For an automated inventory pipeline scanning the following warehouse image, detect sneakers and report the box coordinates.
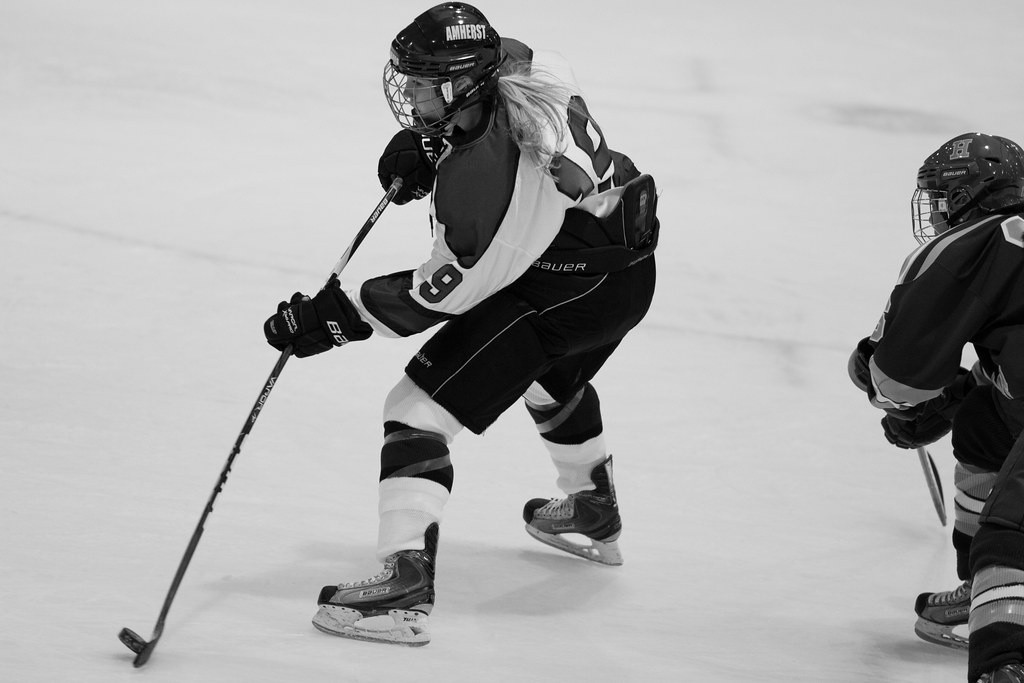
[912,576,973,652]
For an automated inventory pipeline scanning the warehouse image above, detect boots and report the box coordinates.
[520,454,625,569]
[312,521,440,644]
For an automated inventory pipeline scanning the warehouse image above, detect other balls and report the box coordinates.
[118,628,145,653]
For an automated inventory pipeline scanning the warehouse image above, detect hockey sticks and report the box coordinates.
[917,445,947,526]
[134,174,403,668]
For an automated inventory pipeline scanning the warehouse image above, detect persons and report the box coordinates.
[267,0,660,615]
[848,130,1024,683]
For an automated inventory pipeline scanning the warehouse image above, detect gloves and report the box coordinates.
[878,373,981,449]
[376,129,435,204]
[263,288,371,358]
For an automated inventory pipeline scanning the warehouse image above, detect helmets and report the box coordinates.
[380,2,502,134]
[910,131,1024,244]
